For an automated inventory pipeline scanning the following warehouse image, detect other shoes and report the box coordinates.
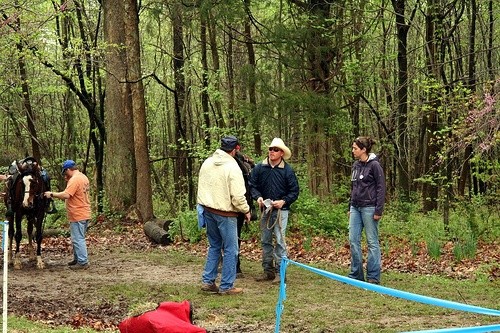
[68,260,77,265]
[218,286,243,296]
[70,263,90,271]
[200,282,218,293]
[272,273,280,283]
[256,272,276,282]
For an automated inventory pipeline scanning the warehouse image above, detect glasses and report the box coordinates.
[269,147,283,153]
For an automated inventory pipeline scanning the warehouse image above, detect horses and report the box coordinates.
[4,159,47,270]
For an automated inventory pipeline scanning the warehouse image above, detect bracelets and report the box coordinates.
[51,192,54,197]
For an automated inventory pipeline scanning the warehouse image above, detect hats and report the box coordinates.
[220,136,240,151]
[61,160,75,175]
[264,138,291,159]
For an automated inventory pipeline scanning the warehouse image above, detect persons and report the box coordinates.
[347,136,386,285]
[248,138,300,285]
[197,136,251,295]
[44,160,92,270]
